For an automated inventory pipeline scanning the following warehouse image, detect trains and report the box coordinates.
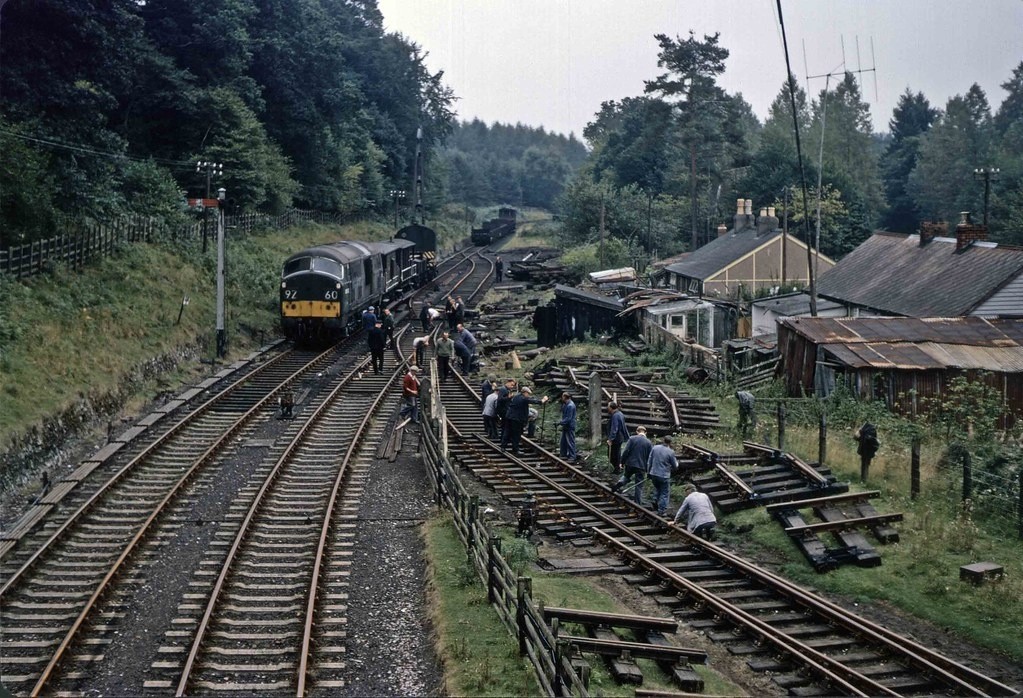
[469,206,518,246]
[277,219,439,353]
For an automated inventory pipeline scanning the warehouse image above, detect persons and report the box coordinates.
[419,306,444,333]
[647,435,679,515]
[495,257,503,283]
[612,425,652,505]
[412,335,429,367]
[607,401,630,474]
[435,331,455,382]
[454,340,471,376]
[400,365,421,424]
[667,483,716,539]
[362,305,395,375]
[446,295,465,333]
[556,391,576,461]
[457,324,477,354]
[481,373,548,455]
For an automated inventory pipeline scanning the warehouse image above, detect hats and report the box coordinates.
[411,366,419,373]
[522,387,532,394]
[369,306,375,310]
[685,484,695,491]
[376,320,383,323]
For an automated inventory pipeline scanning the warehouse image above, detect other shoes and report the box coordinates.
[702,528,708,541]
[710,529,717,541]
[400,413,404,420]
[612,482,623,492]
[412,421,421,424]
